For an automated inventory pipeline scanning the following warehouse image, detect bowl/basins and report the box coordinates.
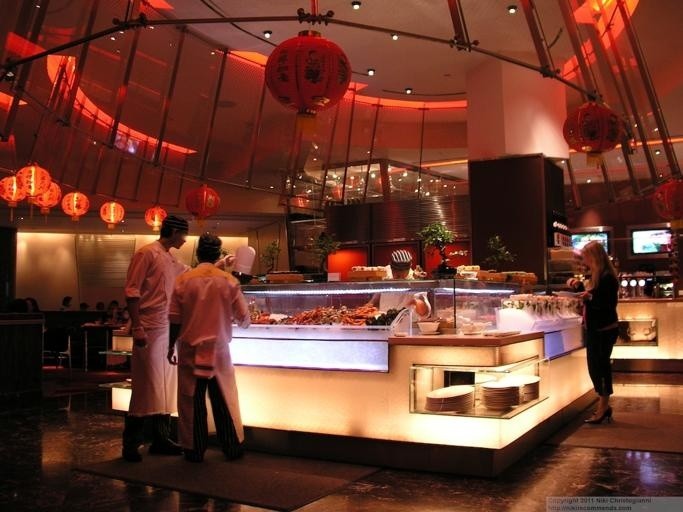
[461,271,478,277]
[418,322,440,332]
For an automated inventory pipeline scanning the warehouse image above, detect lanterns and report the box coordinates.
[61,190,90,222]
[100,201,125,229]
[145,207,167,231]
[650,179,682,221]
[562,98,623,172]
[264,31,351,118]
[15,163,51,201]
[0,175,25,208]
[33,181,61,215]
[184,184,221,227]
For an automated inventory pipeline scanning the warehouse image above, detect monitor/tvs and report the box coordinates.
[571,231,608,258]
[629,227,672,256]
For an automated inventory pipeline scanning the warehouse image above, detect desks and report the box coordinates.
[81,322,155,374]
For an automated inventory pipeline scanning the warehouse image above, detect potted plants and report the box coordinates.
[415,222,458,279]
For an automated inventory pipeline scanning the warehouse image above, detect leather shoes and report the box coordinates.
[122,447,142,461]
[150,440,183,454]
[183,451,202,461]
[227,449,245,462]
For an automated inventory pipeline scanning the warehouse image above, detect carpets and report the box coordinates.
[73,444,382,512]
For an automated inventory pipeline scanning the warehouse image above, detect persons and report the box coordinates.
[363,250,431,323]
[566,240,620,425]
[122,214,236,463]
[168,232,250,461]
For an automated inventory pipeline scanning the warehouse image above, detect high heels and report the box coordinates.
[585,405,613,424]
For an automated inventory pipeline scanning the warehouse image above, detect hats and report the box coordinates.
[391,250,413,271]
[234,246,255,275]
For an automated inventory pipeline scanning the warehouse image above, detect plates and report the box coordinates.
[418,332,440,335]
[482,383,519,409]
[552,291,582,297]
[463,277,478,282]
[519,375,540,403]
[425,385,474,413]
[484,329,522,335]
